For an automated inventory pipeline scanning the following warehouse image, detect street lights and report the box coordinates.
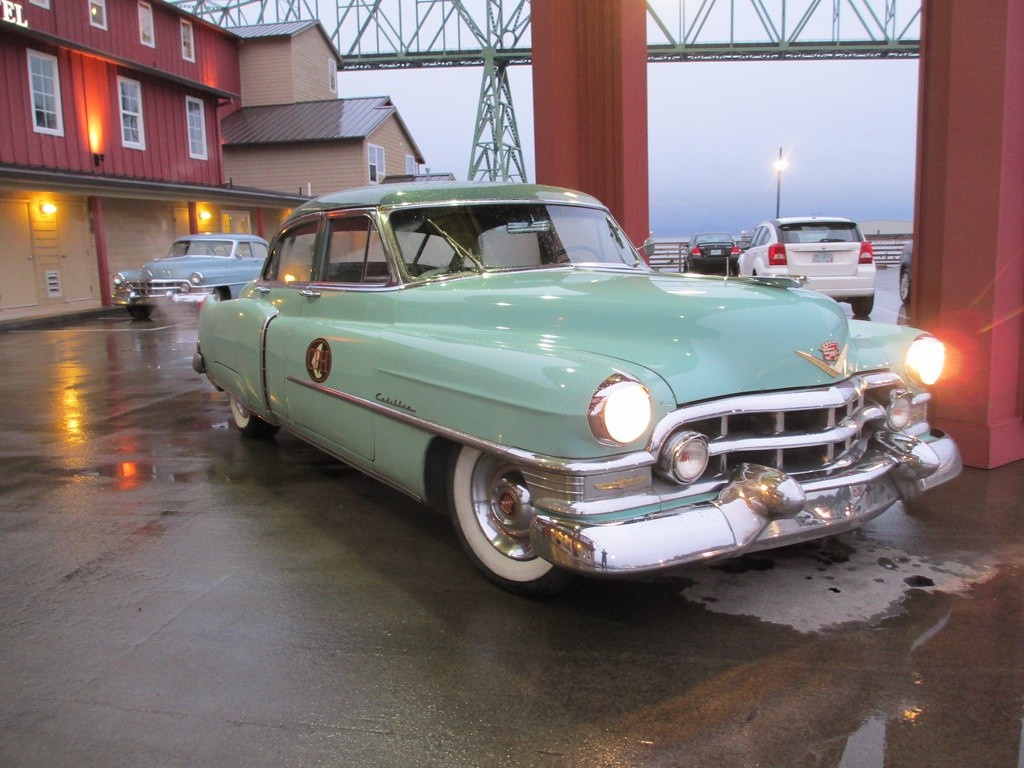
[773,147,792,218]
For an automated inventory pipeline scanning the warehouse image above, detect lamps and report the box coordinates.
[94,152,105,167]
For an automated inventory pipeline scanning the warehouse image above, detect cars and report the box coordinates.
[900,240,914,303]
[111,234,270,319]
[190,180,964,596]
[684,233,740,276]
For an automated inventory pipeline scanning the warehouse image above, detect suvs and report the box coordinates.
[738,215,877,316]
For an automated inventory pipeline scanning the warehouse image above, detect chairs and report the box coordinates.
[826,228,847,241]
[786,232,800,243]
[478,224,541,270]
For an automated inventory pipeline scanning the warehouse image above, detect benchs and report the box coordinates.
[334,261,437,283]
